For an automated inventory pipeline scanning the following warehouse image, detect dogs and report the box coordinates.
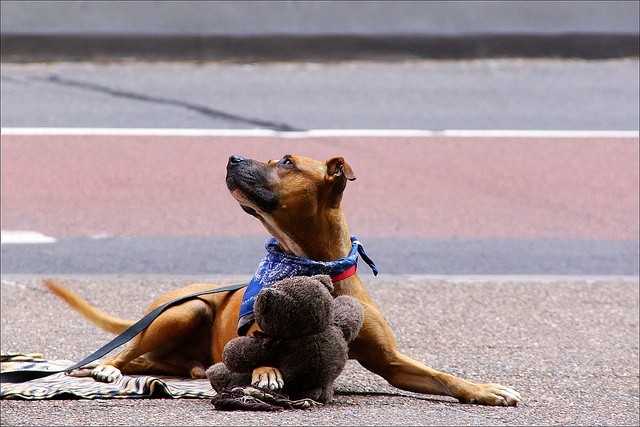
[44,154,523,407]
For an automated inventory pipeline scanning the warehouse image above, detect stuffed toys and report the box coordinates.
[205,274,364,411]
